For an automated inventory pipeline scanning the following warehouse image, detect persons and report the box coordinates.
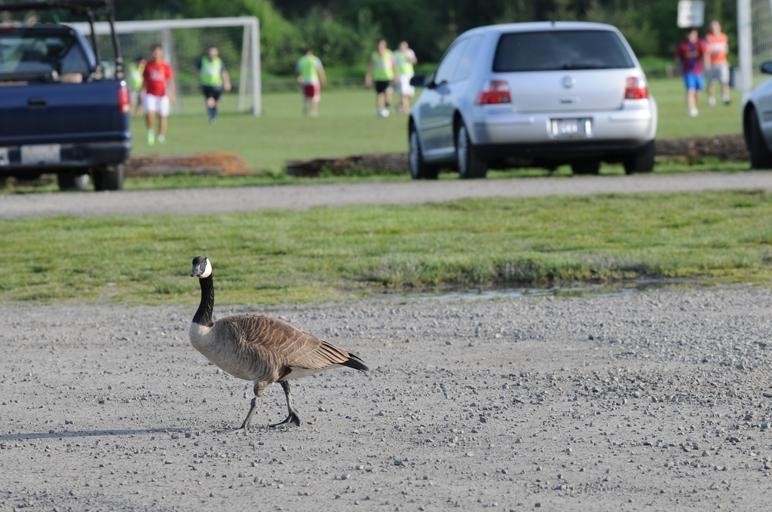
[393,40,418,113]
[126,56,146,118]
[140,44,176,146]
[196,44,232,125]
[675,25,709,116]
[296,48,327,118]
[704,18,731,106]
[365,38,399,118]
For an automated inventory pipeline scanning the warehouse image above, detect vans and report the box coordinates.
[407,21,656,179]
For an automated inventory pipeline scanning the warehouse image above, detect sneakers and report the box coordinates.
[144,128,170,148]
[685,95,738,120]
[370,101,414,118]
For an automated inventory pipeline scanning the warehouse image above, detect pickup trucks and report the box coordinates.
[1,2,129,191]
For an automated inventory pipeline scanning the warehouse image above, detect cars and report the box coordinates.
[743,58,772,170]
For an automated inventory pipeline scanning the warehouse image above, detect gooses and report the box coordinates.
[186,253,368,432]
